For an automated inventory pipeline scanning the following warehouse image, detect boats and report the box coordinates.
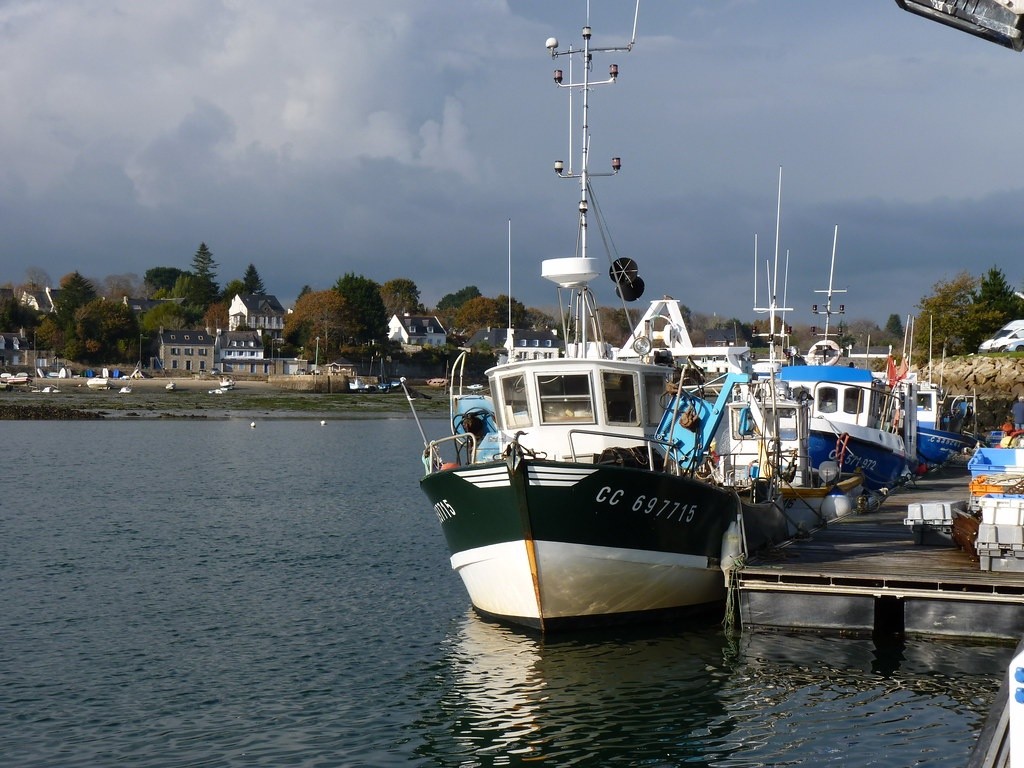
[33,365,149,394]
[426,379,450,387]
[0,377,33,392]
[418,0,977,639]
[349,377,370,393]
[466,384,484,390]
[220,377,236,390]
[210,388,228,394]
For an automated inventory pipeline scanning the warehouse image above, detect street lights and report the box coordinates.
[314,337,320,393]
[140,333,143,373]
[272,339,276,375]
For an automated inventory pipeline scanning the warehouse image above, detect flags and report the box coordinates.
[895,359,908,381]
[888,357,897,388]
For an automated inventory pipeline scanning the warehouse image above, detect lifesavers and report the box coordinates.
[807,340,841,368]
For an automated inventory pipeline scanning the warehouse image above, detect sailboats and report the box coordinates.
[155,356,176,391]
[366,356,402,392]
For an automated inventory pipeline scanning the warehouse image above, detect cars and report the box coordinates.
[296,368,308,375]
[1005,340,1024,353]
[309,369,319,375]
[210,368,222,375]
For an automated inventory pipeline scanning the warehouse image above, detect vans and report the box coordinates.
[978,319,1024,355]
[990,328,1024,353]
